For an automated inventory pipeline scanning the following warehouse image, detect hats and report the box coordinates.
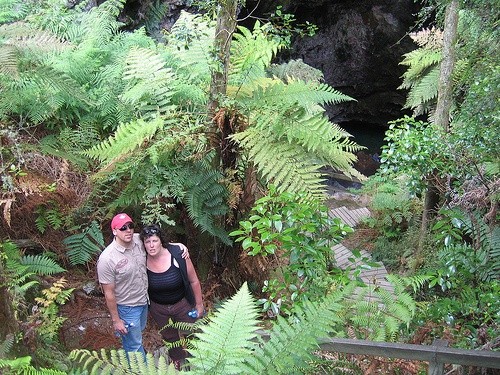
[111,213,134,231]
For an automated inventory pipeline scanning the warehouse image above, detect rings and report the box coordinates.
[186,249,188,250]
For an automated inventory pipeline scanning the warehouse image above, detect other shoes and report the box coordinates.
[174,359,182,371]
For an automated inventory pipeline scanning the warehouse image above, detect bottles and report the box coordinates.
[188,309,205,318]
[113,323,134,338]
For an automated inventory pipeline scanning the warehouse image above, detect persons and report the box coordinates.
[140,224,204,372]
[96,212,189,366]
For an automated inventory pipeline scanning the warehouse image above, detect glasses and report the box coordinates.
[142,229,159,235]
[115,223,135,231]
